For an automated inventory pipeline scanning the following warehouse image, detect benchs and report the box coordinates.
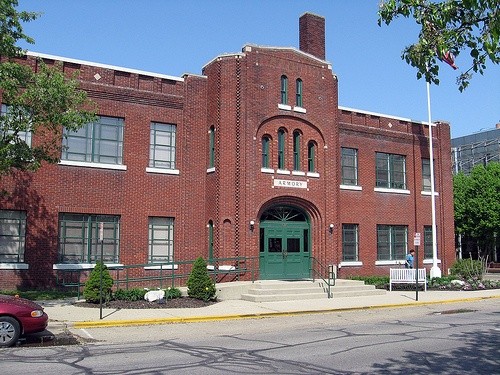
[390,268,427,292]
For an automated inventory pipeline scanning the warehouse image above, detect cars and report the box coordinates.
[0,293,48,350]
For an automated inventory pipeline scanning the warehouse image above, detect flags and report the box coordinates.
[443,51,458,71]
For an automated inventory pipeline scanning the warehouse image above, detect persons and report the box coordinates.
[405,249,415,269]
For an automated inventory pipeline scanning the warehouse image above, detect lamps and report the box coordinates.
[250,220,254,229]
[329,224,334,232]
